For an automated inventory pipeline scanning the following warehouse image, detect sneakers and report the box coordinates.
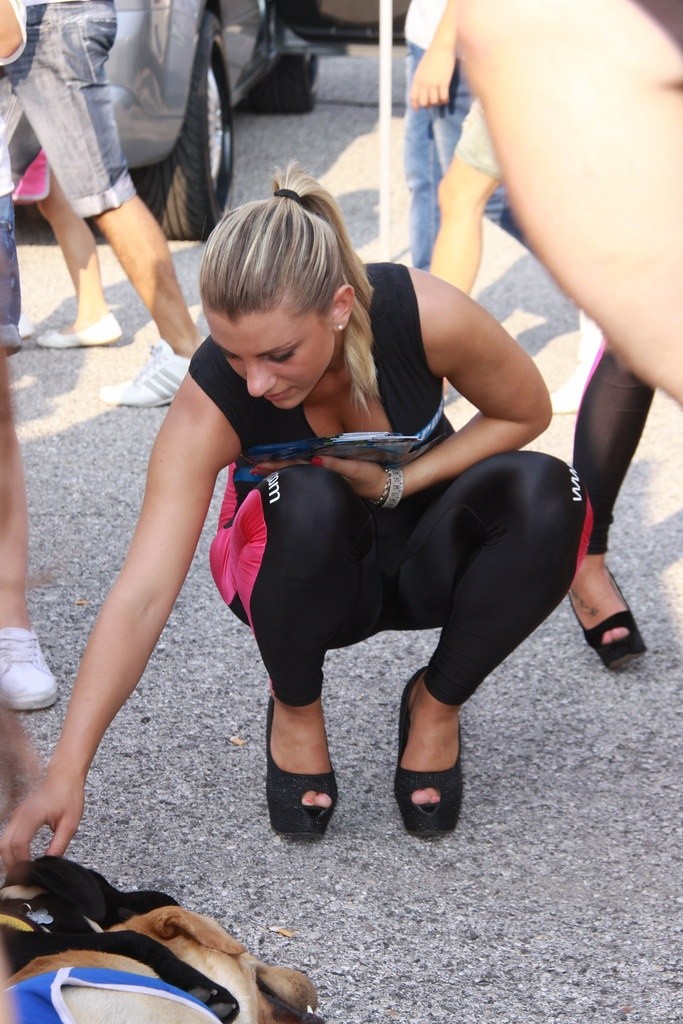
[0,626,58,710]
[99,336,191,407]
[35,312,123,348]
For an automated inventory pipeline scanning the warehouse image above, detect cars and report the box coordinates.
[0,0,322,244]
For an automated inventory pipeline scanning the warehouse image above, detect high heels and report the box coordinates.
[565,563,647,669]
[394,666,463,837]
[267,695,338,838]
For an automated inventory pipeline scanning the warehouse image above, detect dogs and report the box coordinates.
[0,906,319,1024]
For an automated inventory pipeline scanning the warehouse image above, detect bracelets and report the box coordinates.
[371,467,404,509]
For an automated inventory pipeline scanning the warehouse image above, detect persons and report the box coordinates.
[0,0,204,711]
[0,198,594,874]
[404,0,683,670]
